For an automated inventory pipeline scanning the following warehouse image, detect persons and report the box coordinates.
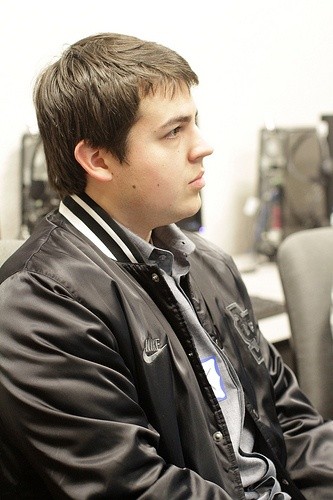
[1,33,333,500]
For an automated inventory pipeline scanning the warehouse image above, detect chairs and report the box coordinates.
[277,226,333,423]
